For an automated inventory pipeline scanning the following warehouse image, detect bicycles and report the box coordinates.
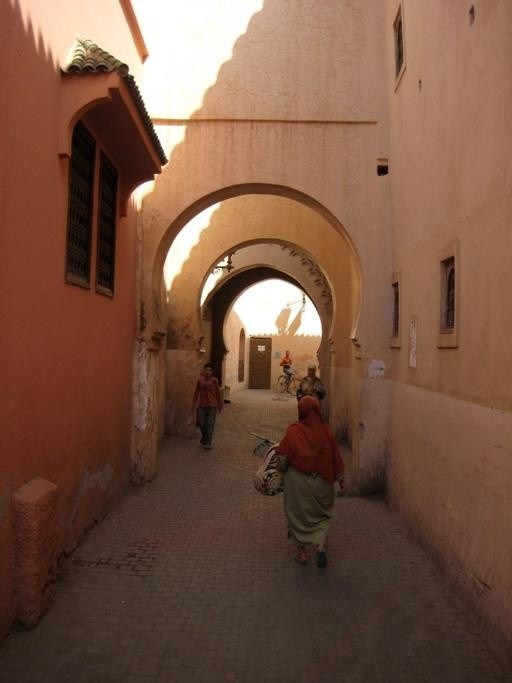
[276,368,303,396]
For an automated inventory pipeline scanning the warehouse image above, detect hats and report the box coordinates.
[308,364,316,370]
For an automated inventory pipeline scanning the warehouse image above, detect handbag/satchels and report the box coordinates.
[253,443,285,497]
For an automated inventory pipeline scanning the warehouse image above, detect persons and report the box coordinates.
[280,349,296,393]
[190,362,224,449]
[275,395,349,568]
[295,362,327,421]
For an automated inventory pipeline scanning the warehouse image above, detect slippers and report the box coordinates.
[295,553,308,564]
[317,550,328,568]
